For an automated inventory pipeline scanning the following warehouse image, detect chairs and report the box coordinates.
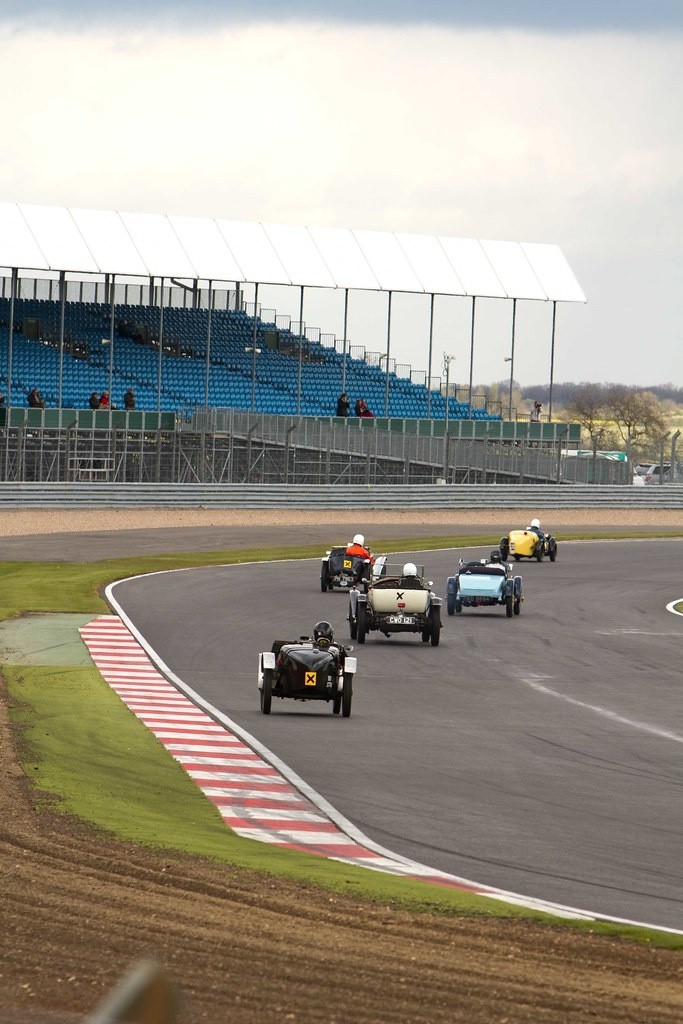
[0,298,503,420]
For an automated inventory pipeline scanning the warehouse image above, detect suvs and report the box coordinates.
[633,462,683,485]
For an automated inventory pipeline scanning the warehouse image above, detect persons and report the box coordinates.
[530,401,543,422]
[89,393,100,409]
[99,391,109,408]
[27,389,44,407]
[124,388,135,409]
[311,622,348,667]
[355,399,374,417]
[0,391,4,407]
[485,551,507,578]
[398,563,420,586]
[528,519,546,542]
[336,393,349,416]
[346,534,371,559]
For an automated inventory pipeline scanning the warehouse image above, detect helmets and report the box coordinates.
[313,621,334,644]
[403,563,417,576]
[353,533,364,547]
[531,519,541,529]
[491,551,501,560]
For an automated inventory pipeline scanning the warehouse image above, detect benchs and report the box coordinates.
[374,577,425,590]
[460,567,506,575]
